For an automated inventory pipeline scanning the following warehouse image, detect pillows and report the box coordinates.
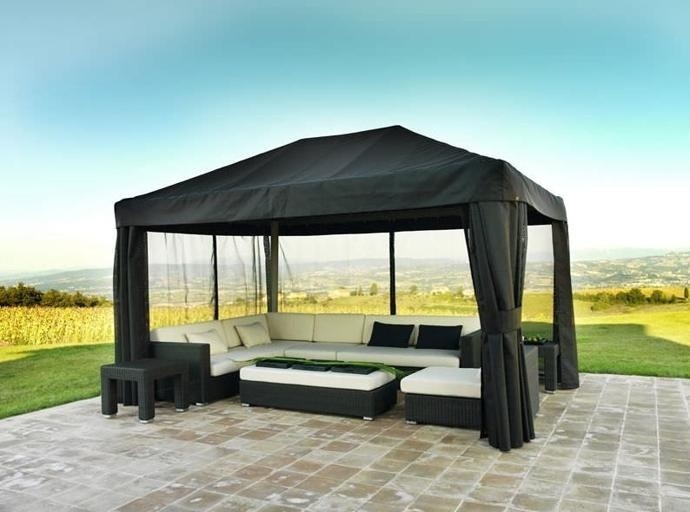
[416,322,463,350]
[234,319,272,348]
[183,328,228,356]
[366,320,416,349]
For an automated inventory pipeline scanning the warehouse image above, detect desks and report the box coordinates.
[97,357,194,424]
[525,338,560,392]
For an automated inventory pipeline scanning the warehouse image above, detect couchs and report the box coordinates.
[148,308,481,407]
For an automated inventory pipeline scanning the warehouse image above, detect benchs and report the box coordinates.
[237,359,398,423]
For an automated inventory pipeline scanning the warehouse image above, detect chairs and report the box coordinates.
[399,346,543,429]
